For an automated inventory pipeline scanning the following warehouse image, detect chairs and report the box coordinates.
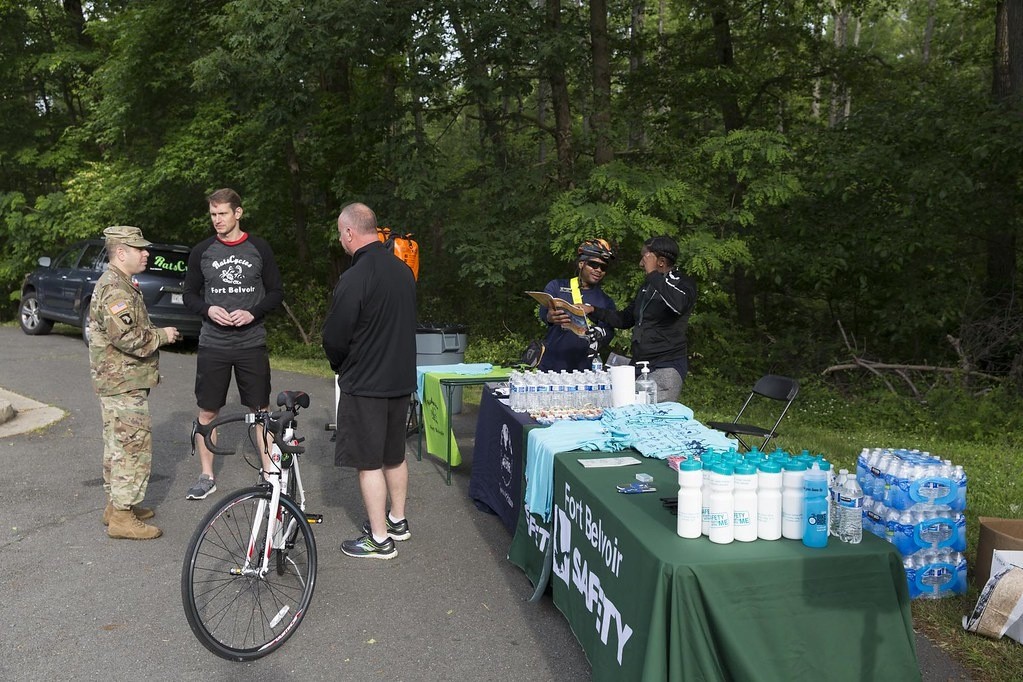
[707,373,802,450]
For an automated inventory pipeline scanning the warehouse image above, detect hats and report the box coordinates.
[103,226,153,248]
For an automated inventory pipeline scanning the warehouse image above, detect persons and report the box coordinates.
[321,202,418,561]
[571,235,698,405]
[534,236,618,376]
[182,187,285,500]
[87,226,180,540]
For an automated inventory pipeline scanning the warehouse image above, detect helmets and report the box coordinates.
[577,238,616,264]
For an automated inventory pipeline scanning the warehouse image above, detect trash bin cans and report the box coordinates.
[408,328,467,414]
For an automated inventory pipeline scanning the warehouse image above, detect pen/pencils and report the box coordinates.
[660,498,678,515]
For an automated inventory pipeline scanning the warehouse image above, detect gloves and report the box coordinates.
[585,325,606,358]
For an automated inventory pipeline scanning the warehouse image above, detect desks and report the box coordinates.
[522,423,923,682]
[472,382,601,535]
[414,363,515,485]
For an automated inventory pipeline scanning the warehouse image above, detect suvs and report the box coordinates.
[17,237,203,339]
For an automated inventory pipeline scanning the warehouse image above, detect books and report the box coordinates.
[525,290,591,338]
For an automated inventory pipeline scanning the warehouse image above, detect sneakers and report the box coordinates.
[186,473,217,500]
[363,510,411,541]
[257,466,271,486]
[340,532,398,560]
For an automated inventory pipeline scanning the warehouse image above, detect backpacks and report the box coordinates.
[376,227,419,283]
[501,340,545,372]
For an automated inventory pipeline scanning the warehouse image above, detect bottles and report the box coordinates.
[591,353,604,371]
[676,447,836,549]
[831,470,851,535]
[839,473,862,544]
[854,447,970,599]
[509,369,613,413]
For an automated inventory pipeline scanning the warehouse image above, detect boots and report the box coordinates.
[102,494,154,525]
[108,508,163,540]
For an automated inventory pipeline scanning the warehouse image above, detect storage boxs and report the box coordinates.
[977,516,1023,599]
[982,550,1023,644]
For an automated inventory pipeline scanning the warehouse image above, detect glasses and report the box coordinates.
[585,259,608,272]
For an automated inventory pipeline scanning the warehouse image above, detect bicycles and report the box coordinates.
[180,390,324,667]
